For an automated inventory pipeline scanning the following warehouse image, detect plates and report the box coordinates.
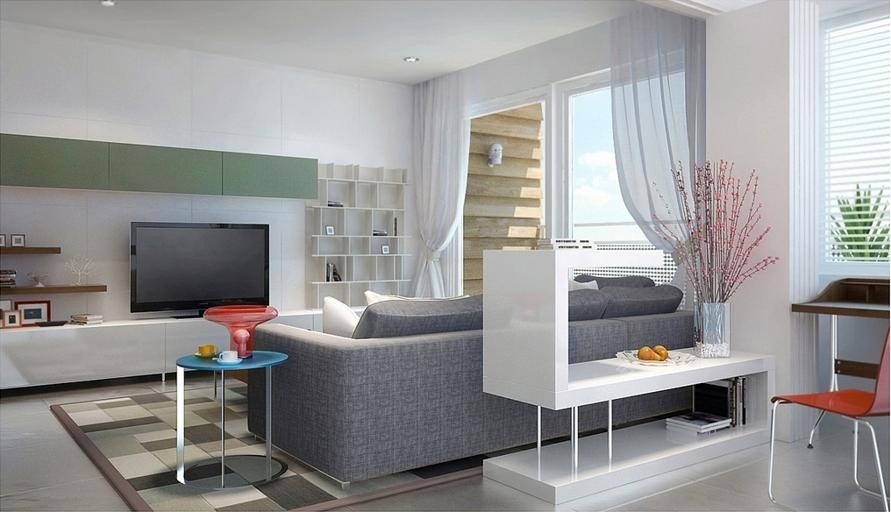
[194,351,219,357]
[217,359,242,364]
[616,351,697,371]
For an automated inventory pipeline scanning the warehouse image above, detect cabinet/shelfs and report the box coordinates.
[0,307,365,392]
[305,159,417,309]
[0,234,108,296]
[483,247,775,504]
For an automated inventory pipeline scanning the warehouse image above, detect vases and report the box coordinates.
[692,304,731,358]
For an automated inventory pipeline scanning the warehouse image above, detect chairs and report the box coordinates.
[768,324,889,512]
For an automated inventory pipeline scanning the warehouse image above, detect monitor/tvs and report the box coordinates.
[129,221,269,319]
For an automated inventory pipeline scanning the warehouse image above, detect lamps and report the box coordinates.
[488,143,504,168]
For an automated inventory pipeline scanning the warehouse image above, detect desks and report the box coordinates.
[792,278,891,450]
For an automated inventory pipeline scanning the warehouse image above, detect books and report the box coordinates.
[664,411,733,436]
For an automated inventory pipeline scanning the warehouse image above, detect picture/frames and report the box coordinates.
[381,244,391,254]
[325,225,335,235]
[1,233,7,246]
[3,312,20,328]
[15,301,51,326]
[11,234,27,247]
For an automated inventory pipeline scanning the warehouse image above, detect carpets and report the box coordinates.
[49,379,692,512]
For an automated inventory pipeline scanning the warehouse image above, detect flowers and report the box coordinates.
[647,159,781,342]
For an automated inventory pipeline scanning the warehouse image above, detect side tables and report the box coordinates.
[176,350,290,492]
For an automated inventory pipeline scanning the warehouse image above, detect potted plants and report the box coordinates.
[830,184,889,261]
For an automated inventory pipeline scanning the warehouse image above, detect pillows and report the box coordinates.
[567,277,599,291]
[364,290,471,306]
[321,296,359,337]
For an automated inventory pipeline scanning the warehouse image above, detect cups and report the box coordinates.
[219,351,238,362]
[198,345,213,354]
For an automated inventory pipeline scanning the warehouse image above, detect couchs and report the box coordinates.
[248,273,696,490]
